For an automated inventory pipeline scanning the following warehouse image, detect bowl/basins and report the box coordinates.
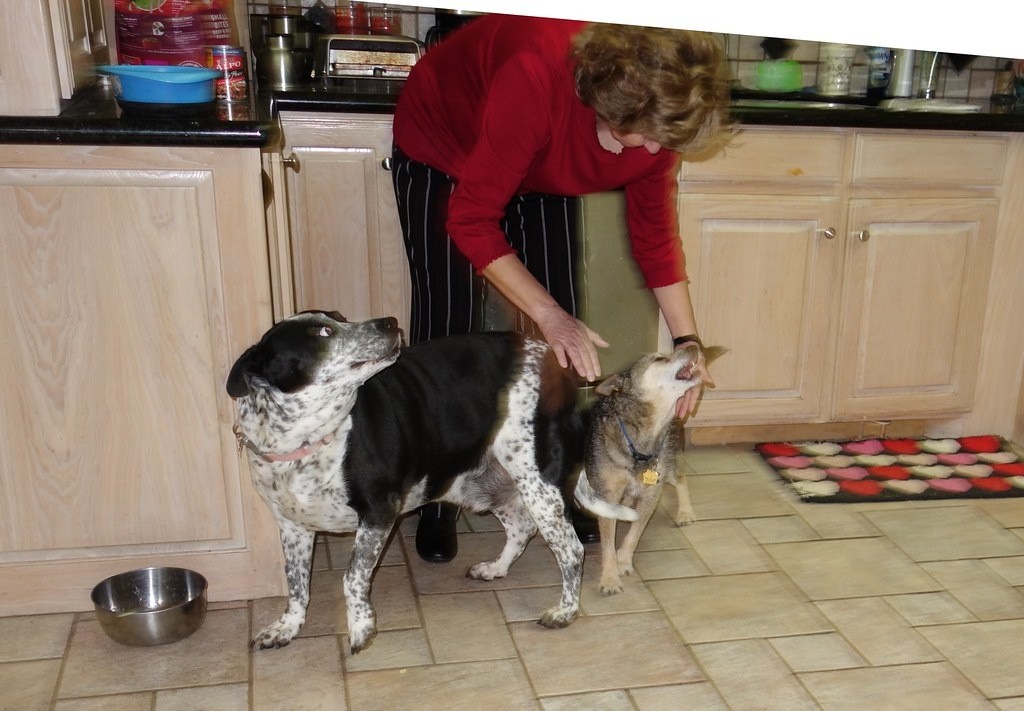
[90,566,208,651]
[94,63,224,106]
[1011,78,1023,106]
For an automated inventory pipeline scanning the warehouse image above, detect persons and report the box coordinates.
[391,13,741,563]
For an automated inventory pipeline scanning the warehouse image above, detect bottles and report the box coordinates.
[868,48,941,101]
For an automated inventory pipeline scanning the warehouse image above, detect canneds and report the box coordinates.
[211,46,249,103]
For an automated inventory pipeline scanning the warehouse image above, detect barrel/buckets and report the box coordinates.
[258,33,313,93]
[250,14,318,60]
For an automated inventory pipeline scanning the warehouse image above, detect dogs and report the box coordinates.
[225,309,641,655]
[586,344,730,596]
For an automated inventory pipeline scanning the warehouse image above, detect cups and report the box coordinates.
[267,1,299,13]
[335,1,368,33]
[820,45,856,95]
[370,4,401,34]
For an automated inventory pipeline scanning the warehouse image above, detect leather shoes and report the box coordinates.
[415,517,458,562]
[570,505,600,544]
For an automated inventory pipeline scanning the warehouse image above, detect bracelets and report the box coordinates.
[673,333,704,352]
